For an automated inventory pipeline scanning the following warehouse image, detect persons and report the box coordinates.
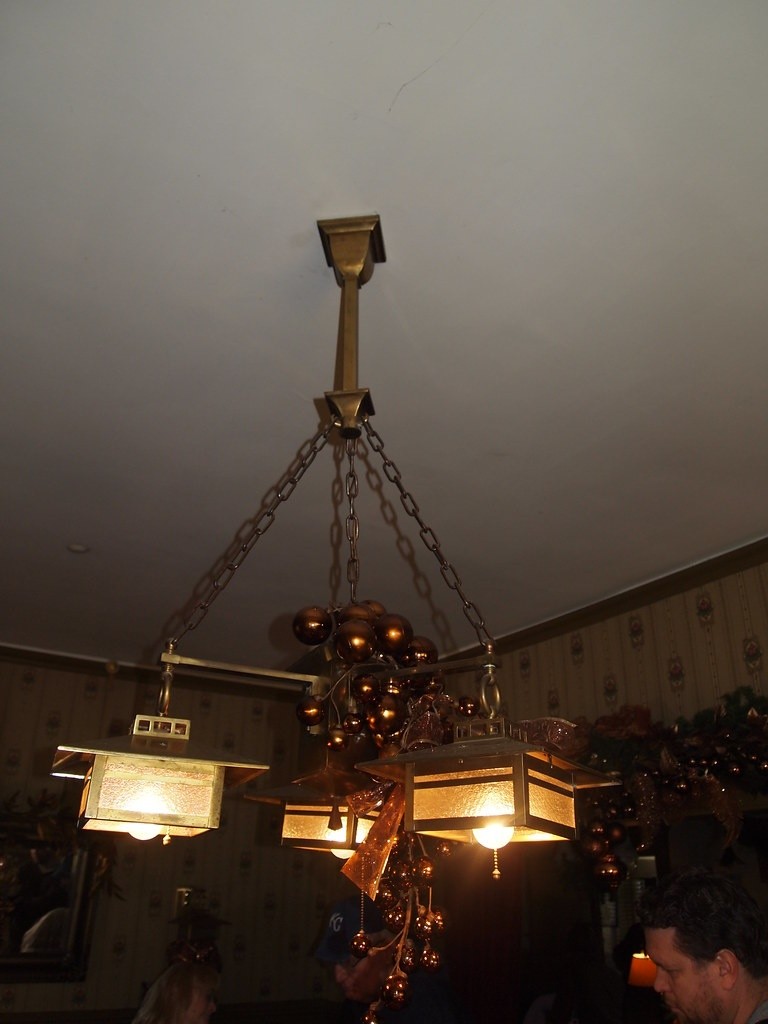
[307,895,468,1024]
[132,960,221,1024]
[635,864,768,1024]
[8,847,71,954]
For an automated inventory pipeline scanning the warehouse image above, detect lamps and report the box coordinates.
[628,952,659,988]
[48,211,623,1023]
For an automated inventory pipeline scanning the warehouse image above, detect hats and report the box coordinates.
[312,896,395,967]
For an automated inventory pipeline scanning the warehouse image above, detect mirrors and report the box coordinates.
[0,789,114,984]
